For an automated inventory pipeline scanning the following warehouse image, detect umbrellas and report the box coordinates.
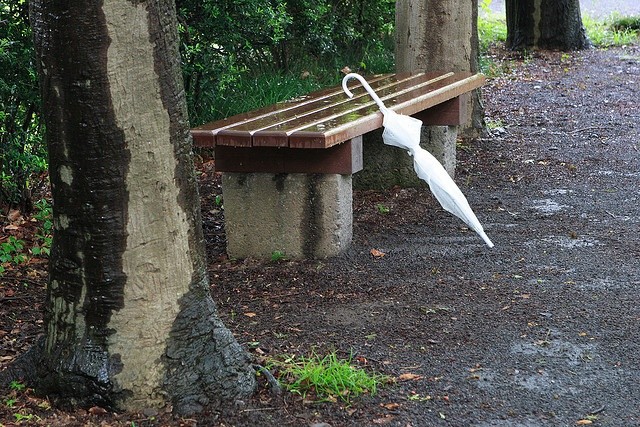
[342,72,495,248]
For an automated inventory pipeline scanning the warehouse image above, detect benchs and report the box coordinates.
[188,71,486,262]
